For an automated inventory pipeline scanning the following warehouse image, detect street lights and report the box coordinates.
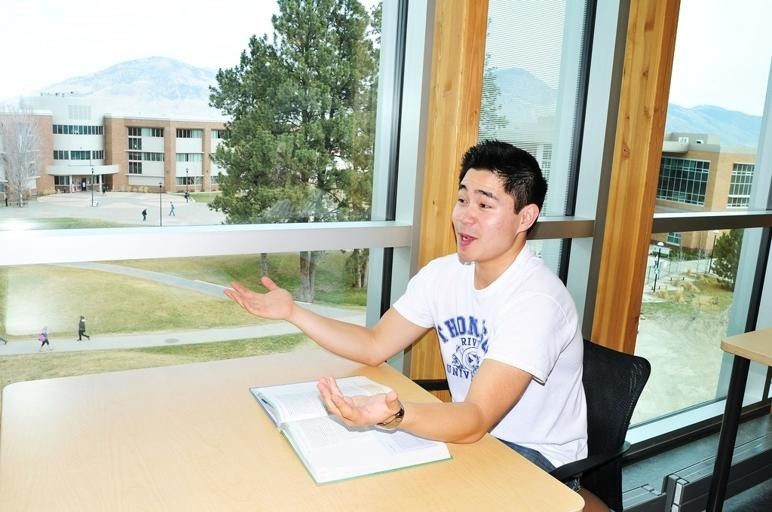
[91,169,94,206]
[652,241,664,291]
[185,168,189,202]
[708,230,719,273]
[158,182,163,227]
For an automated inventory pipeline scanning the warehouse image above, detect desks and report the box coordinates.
[706,325,772,511]
[0,347,584,511]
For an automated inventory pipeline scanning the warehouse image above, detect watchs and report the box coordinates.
[378,407,406,431]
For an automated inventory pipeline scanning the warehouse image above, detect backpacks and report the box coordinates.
[38,334,46,342]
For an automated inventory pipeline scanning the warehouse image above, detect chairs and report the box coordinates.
[411,336,651,511]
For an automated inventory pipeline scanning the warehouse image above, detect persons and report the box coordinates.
[76,315,90,342]
[0,335,8,345]
[223,138,592,497]
[141,208,147,221]
[169,201,175,216]
[184,191,190,203]
[39,324,54,353]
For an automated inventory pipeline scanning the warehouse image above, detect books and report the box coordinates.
[247,374,452,486]
